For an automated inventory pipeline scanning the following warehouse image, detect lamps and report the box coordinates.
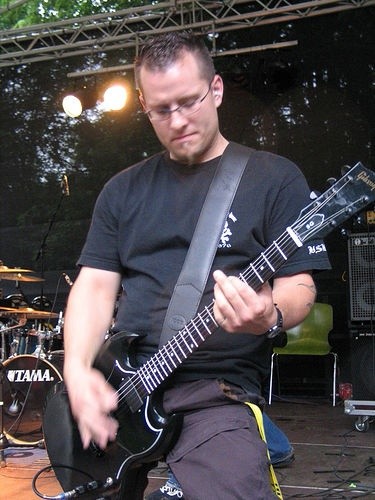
[62,73,97,119]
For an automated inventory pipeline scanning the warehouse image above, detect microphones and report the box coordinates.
[61,273,73,287]
[62,174,71,198]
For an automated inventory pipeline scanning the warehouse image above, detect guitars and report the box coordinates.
[41,161,375,500]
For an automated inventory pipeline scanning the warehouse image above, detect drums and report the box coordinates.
[9,328,64,360]
[0,354,64,446]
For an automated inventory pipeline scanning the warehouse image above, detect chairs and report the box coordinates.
[268,302,337,406]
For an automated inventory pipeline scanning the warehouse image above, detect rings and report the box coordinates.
[218,317,226,325]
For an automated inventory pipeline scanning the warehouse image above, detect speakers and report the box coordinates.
[346,231,374,322]
[350,319,375,402]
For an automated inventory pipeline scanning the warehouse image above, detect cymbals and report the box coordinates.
[0,307,58,319]
[0,265,34,273]
[0,274,47,282]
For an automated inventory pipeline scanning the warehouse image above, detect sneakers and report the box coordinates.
[148,483,183,500]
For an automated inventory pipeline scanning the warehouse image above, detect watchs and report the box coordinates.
[264,304,283,338]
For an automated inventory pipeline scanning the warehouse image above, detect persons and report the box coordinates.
[65,30,334,499]
[145,412,294,500]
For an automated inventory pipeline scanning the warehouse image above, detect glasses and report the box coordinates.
[140,80,213,121]
